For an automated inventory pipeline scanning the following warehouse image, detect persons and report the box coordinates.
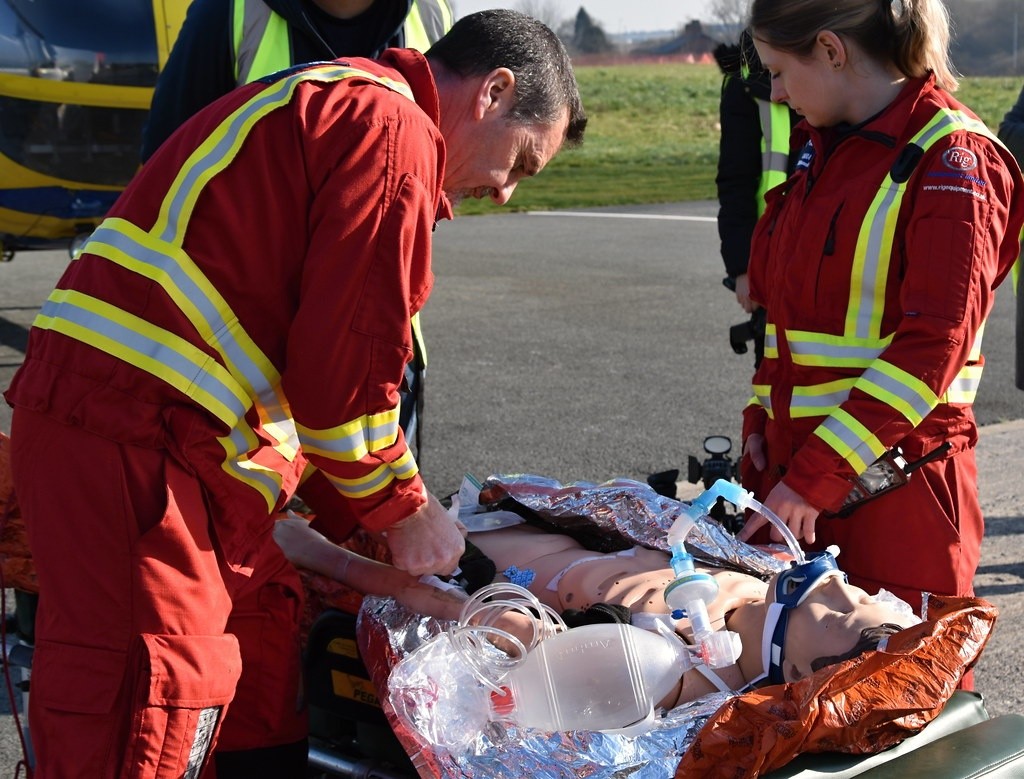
[997,87,1024,392]
[272,505,923,712]
[6,10,591,779]
[715,25,801,369]
[734,0,1024,689]
[135,1,452,473]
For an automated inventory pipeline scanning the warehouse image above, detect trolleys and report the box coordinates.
[1,590,1024,779]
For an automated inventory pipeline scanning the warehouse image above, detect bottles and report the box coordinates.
[485,623,743,731]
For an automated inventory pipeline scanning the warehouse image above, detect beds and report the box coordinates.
[0,583,1024,779]
